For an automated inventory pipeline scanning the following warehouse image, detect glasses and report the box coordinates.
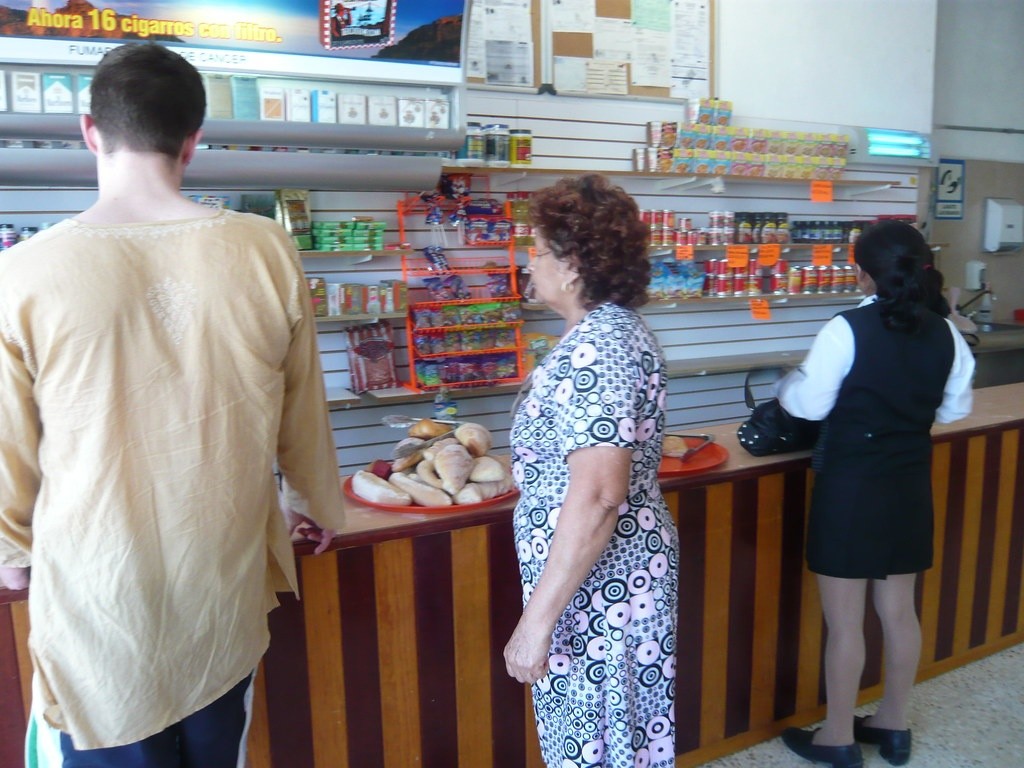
[531,249,552,257]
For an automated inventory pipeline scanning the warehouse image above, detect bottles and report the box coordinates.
[980,291,993,333]
[504,190,536,247]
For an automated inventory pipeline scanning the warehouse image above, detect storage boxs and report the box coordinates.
[633,98,851,179]
[273,188,386,251]
[307,277,408,317]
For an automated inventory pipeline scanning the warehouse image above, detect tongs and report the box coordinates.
[379,415,466,459]
[661,431,715,464]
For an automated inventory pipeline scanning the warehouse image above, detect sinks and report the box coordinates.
[974,321,1024,334]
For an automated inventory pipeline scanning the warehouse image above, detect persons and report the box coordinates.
[775,213,977,766]
[0,41,351,768]
[504,175,683,768]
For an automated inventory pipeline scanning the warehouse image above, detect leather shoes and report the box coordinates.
[853,715,911,766]
[782,727,863,768]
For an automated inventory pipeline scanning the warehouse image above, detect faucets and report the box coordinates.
[958,290,997,311]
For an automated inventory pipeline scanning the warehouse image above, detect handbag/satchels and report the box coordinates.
[737,367,822,457]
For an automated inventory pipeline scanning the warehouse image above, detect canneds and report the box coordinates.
[637,209,790,244]
[791,220,871,245]
[702,258,859,294]
[454,122,535,165]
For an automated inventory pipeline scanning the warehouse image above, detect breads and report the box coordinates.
[350,419,514,507]
[662,435,688,457]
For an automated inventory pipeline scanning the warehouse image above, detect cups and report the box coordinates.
[0,224,38,253]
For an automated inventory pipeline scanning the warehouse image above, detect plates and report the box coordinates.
[656,437,729,478]
[343,466,518,514]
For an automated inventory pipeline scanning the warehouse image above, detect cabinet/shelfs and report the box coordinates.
[395,198,525,395]
[299,248,413,409]
[366,164,901,404]
